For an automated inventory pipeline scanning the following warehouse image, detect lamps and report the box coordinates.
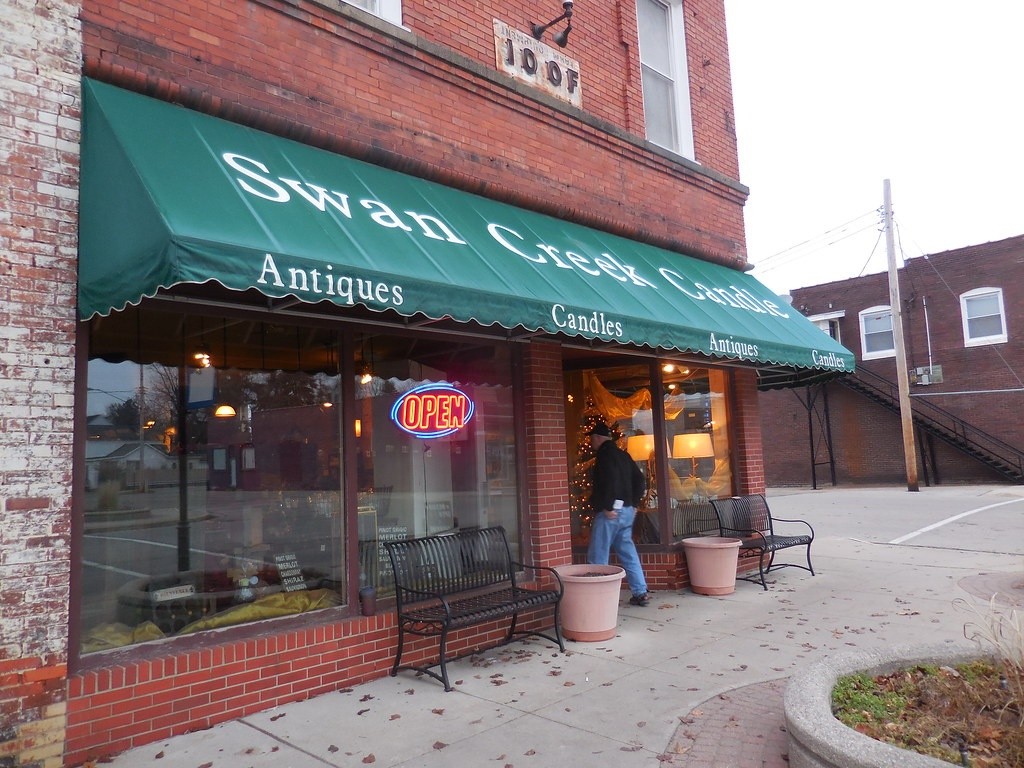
[672,433,715,480]
[532,0,573,48]
[214,308,237,417]
[628,434,673,508]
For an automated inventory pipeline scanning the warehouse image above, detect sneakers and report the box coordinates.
[630,592,649,606]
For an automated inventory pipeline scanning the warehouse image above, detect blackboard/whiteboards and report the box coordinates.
[273,552,308,592]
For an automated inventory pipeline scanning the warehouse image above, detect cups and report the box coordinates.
[361,587,377,616]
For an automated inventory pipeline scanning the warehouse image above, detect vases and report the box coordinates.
[551,564,627,642]
[681,536,743,596]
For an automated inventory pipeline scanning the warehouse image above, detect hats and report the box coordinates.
[584,423,611,437]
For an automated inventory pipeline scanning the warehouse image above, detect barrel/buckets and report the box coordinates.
[552,565,627,642]
[683,538,743,595]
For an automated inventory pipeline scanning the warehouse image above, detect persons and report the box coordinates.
[584,424,652,605]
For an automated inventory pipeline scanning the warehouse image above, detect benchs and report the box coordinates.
[383,526,566,693]
[709,494,815,591]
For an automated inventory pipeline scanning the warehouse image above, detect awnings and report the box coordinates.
[80,78,856,391]
